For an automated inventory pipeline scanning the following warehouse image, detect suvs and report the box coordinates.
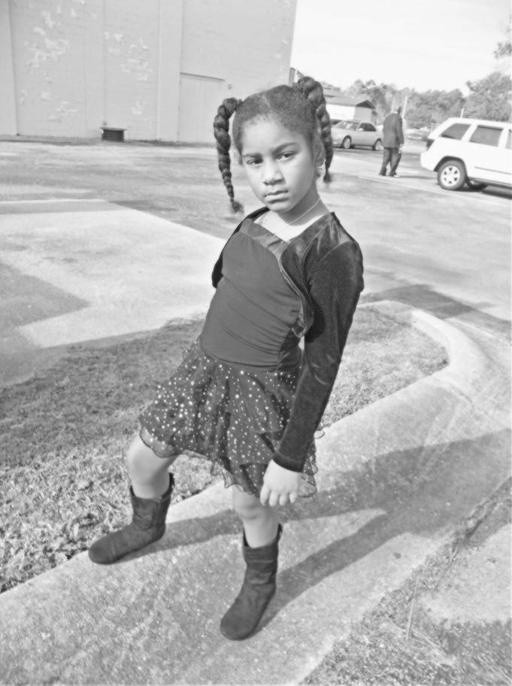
[420,115,512,193]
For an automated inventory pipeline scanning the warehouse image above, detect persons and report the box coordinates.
[378,106,404,177]
[88,77,364,641]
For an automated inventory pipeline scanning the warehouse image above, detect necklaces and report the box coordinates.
[263,197,321,233]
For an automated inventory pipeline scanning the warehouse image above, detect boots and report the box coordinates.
[220,524,282,640]
[88,474,174,563]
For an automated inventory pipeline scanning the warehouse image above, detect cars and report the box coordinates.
[331,118,384,150]
